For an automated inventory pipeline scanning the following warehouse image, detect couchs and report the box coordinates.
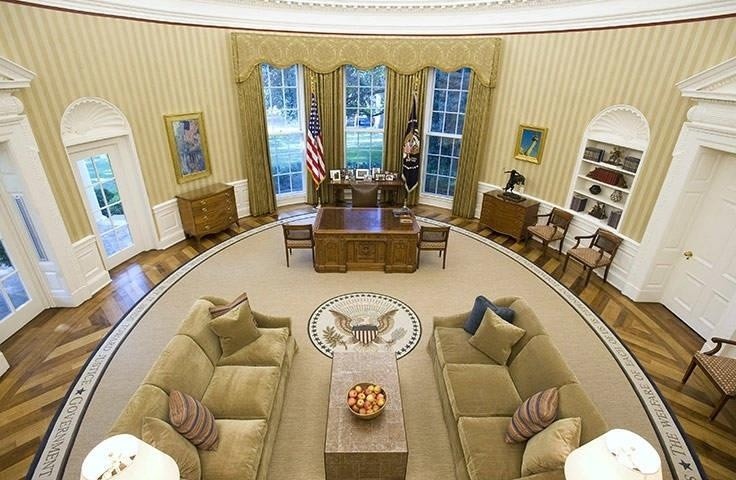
[426,295,608,480]
[103,294,299,480]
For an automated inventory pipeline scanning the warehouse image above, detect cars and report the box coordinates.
[347,116,374,127]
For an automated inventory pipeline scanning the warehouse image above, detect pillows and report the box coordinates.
[504,386,559,445]
[520,417,582,477]
[208,300,262,358]
[168,387,219,450]
[209,291,259,327]
[468,307,527,366]
[462,295,515,335]
[141,416,202,480]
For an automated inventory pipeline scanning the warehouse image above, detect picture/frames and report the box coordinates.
[329,169,397,182]
[513,124,549,165]
[163,111,212,184]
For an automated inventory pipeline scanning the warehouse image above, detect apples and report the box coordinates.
[347,383,384,414]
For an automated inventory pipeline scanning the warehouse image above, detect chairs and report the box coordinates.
[525,207,574,256]
[682,336,736,422]
[563,228,624,286]
[281,223,315,267]
[417,225,450,269]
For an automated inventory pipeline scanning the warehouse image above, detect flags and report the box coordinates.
[304,91,327,192]
[400,88,420,194]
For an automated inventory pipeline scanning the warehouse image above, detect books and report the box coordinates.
[583,146,605,162]
[607,210,622,229]
[571,195,588,212]
[623,156,640,174]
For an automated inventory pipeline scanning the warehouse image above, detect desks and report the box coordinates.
[326,178,404,207]
[312,207,420,273]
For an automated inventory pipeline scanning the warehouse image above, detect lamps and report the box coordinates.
[80,433,180,480]
[564,428,663,480]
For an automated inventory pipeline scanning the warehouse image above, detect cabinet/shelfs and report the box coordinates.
[569,139,644,230]
[478,190,541,244]
[175,182,240,245]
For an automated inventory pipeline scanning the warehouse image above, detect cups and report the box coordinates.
[345,175,349,183]
